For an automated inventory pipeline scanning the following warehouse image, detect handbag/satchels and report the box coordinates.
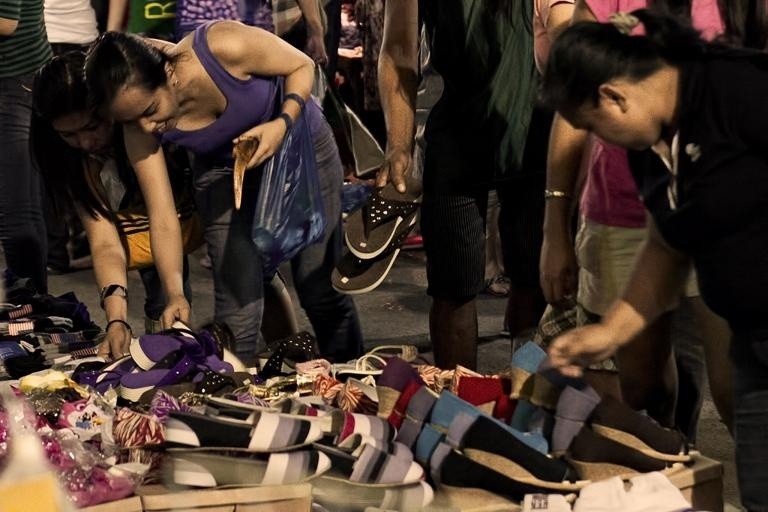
[327,84,387,179]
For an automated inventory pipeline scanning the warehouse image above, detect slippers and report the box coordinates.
[342,176,423,262]
[330,248,403,296]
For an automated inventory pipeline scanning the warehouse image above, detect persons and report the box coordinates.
[0,1,768,512]
